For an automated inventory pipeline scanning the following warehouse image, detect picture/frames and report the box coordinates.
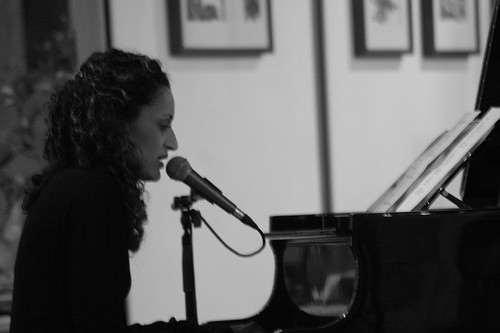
[421,0,480,56]
[167,0,274,55]
[354,0,414,54]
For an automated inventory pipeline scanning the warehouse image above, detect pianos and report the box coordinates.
[204,1,500,333]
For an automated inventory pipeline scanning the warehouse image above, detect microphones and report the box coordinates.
[166,157,258,229]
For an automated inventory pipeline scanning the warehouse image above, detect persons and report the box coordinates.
[9,49,186,333]
